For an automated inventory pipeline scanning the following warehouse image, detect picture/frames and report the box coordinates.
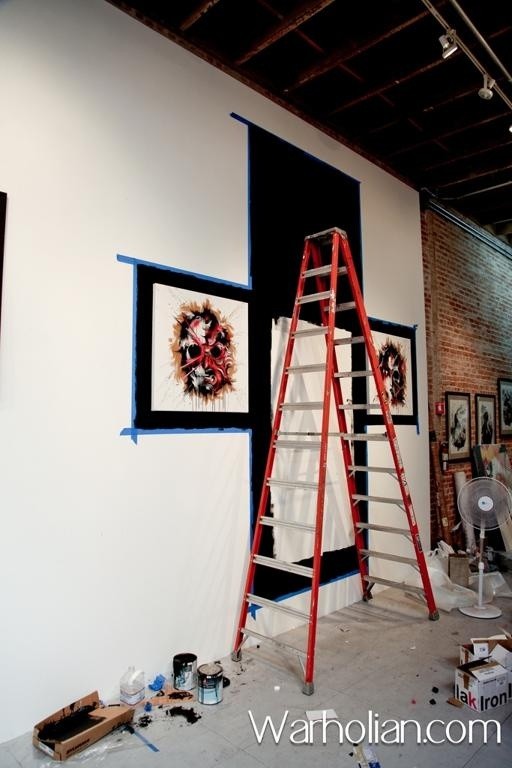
[358,315,424,428]
[133,263,261,430]
[440,375,510,467]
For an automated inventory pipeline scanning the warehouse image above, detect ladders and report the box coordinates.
[230,227,439,695]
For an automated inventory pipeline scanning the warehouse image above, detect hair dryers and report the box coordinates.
[453,471,476,554]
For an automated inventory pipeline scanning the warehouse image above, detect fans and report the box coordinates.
[455,473,512,621]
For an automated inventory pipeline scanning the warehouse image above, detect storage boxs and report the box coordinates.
[451,626,511,714]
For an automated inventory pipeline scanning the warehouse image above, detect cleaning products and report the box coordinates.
[120,662,146,715]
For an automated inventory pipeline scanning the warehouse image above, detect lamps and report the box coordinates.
[439,27,459,59]
[477,72,497,101]
